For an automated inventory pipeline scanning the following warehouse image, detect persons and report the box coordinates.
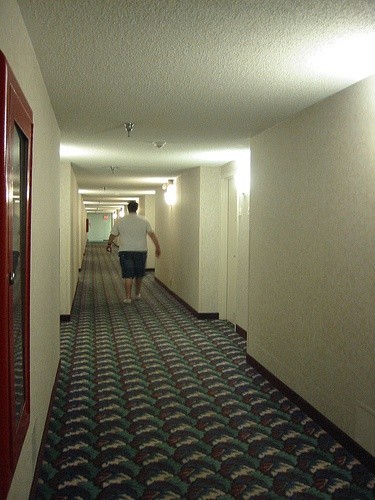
[106,201,161,304]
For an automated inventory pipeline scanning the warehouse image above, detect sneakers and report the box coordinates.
[123,299,131,303]
[135,293,141,300]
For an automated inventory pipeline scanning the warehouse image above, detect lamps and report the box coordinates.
[124,123,134,139]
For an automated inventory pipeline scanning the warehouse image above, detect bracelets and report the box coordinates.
[108,243,111,245]
